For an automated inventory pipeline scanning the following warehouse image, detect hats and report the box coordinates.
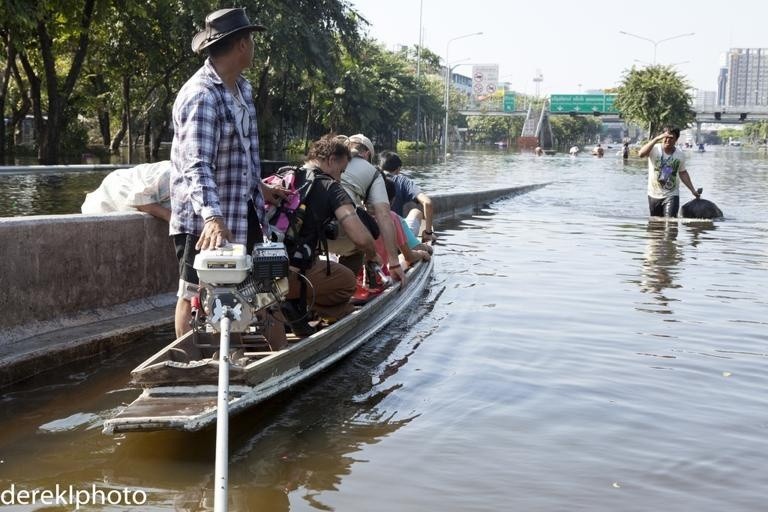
[192,7,268,54]
[344,133,374,163]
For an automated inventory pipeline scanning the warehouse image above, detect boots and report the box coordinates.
[339,254,380,304]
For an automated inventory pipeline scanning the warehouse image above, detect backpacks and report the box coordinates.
[254,165,334,270]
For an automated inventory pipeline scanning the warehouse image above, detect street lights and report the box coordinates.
[619,29,697,71]
[633,58,690,72]
[443,32,483,155]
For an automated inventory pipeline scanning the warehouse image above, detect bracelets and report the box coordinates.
[425,229,433,235]
[389,264,401,270]
[203,215,228,224]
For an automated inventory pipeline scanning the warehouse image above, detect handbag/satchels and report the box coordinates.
[358,206,380,239]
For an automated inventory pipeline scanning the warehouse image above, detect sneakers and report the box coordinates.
[279,299,316,338]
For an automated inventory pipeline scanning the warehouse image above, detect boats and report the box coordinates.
[103,219,434,433]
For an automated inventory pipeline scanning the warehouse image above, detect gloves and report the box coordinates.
[194,215,234,251]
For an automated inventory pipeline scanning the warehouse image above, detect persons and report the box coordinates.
[638,124,700,218]
[168,7,295,338]
[80,157,171,222]
[366,178,431,274]
[261,133,378,337]
[398,208,433,255]
[569,145,580,156]
[622,142,630,158]
[376,149,437,246]
[317,131,408,291]
[592,144,604,158]
[534,143,544,156]
